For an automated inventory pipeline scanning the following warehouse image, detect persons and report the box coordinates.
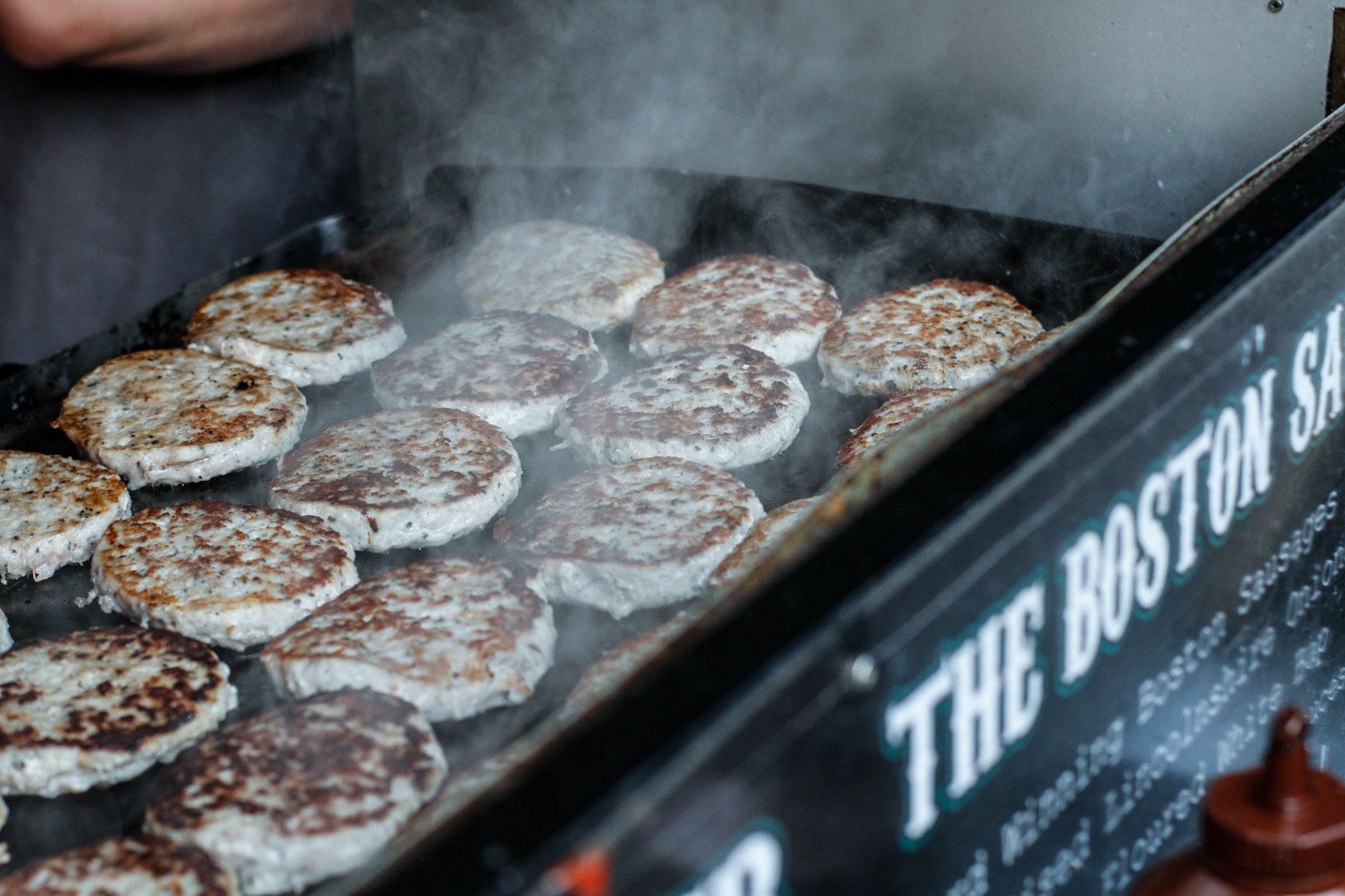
[1,0,357,74]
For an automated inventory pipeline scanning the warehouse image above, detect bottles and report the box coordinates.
[1123,706,1345,896]
